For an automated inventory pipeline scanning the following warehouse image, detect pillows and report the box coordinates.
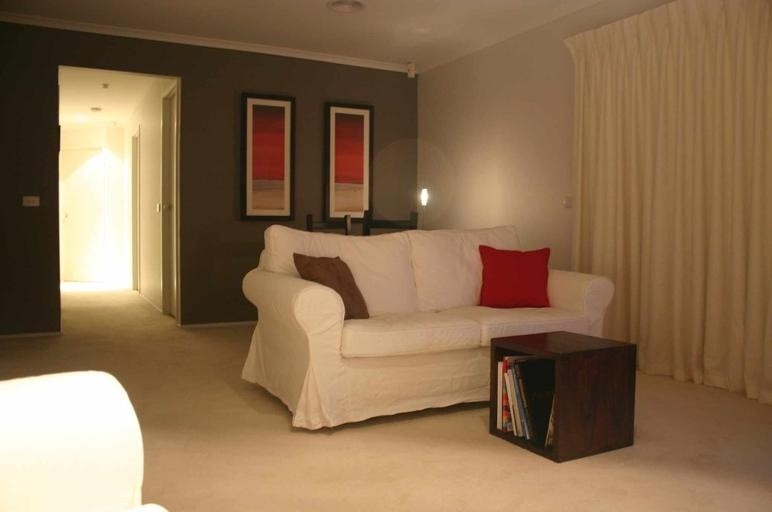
[294,254,369,319]
[478,245,550,308]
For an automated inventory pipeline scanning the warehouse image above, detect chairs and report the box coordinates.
[306,214,351,235]
[0,369,169,512]
[362,210,418,236]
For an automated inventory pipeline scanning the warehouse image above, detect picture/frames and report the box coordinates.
[240,90,296,220]
[322,102,375,228]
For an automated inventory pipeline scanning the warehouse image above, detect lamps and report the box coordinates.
[419,188,430,230]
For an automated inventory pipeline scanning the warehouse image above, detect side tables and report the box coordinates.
[489,332,637,462]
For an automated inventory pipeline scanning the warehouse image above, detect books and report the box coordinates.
[495,355,553,449]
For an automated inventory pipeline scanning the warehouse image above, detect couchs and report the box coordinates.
[241,225,614,431]
